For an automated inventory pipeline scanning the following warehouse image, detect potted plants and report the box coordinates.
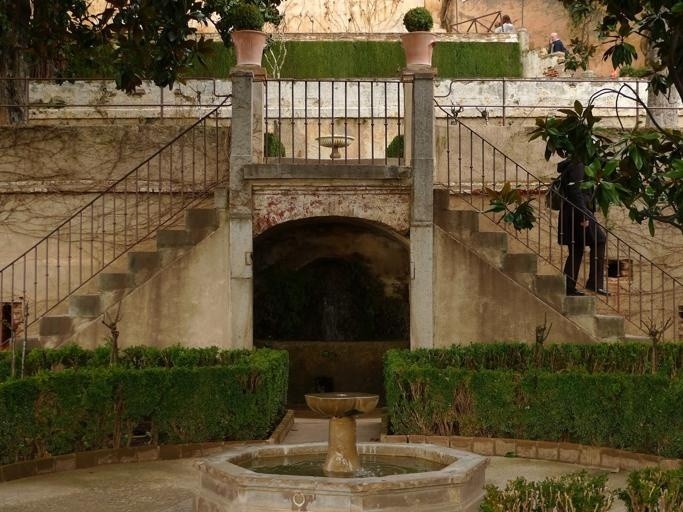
[401,7,436,68]
[232,3,265,69]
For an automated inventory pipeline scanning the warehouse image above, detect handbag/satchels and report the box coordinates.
[546,181,563,210]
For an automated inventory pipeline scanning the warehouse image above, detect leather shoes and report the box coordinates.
[569,290,585,296]
[586,284,611,296]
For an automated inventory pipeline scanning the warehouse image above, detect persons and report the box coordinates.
[557,155,614,296]
[548,33,565,54]
[495,14,514,33]
